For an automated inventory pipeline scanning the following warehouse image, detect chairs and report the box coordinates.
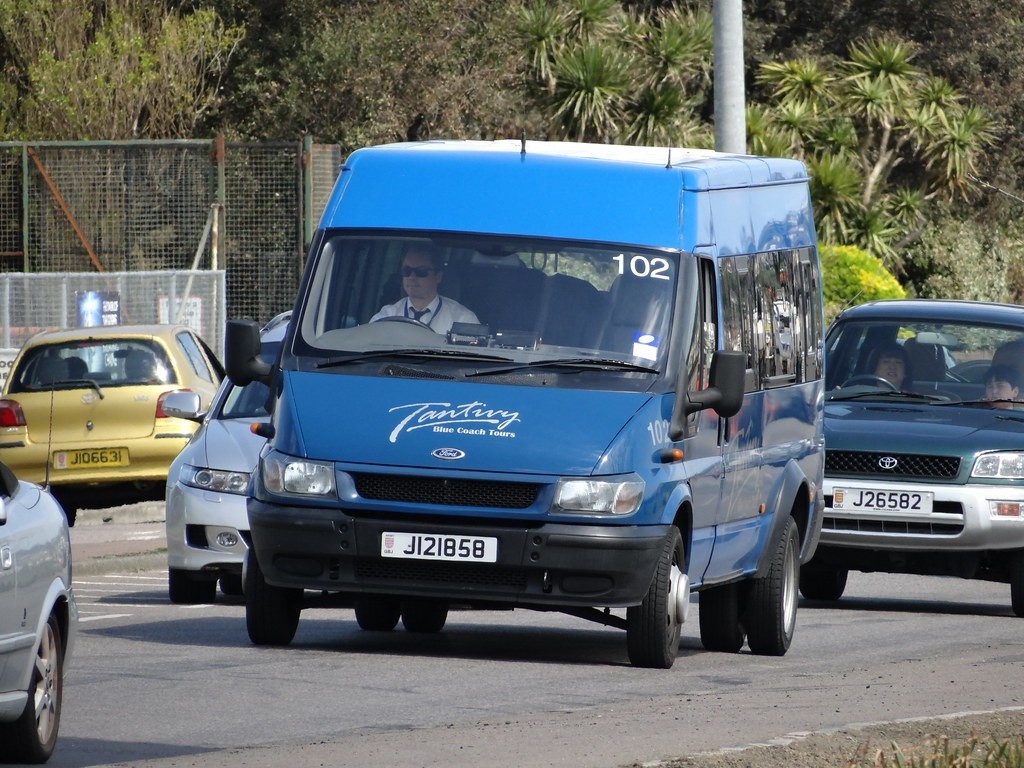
[399,270,462,302]
[904,337,945,382]
[588,273,671,353]
[125,348,154,378]
[65,356,88,379]
[992,341,1024,392]
[36,355,68,384]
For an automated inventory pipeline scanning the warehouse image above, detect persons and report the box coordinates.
[857,340,917,394]
[979,362,1024,412]
[370,241,479,338]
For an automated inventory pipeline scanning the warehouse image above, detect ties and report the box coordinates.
[410,307,430,321]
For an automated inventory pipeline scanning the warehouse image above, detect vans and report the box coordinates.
[221,136,829,670]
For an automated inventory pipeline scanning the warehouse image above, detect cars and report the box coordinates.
[0,461,77,768]
[156,307,355,610]
[0,319,222,487]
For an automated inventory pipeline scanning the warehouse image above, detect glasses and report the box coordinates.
[402,264,439,278]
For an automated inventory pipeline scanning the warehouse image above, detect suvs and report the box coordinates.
[799,298,1024,618]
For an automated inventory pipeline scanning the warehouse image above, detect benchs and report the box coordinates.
[452,263,611,347]
